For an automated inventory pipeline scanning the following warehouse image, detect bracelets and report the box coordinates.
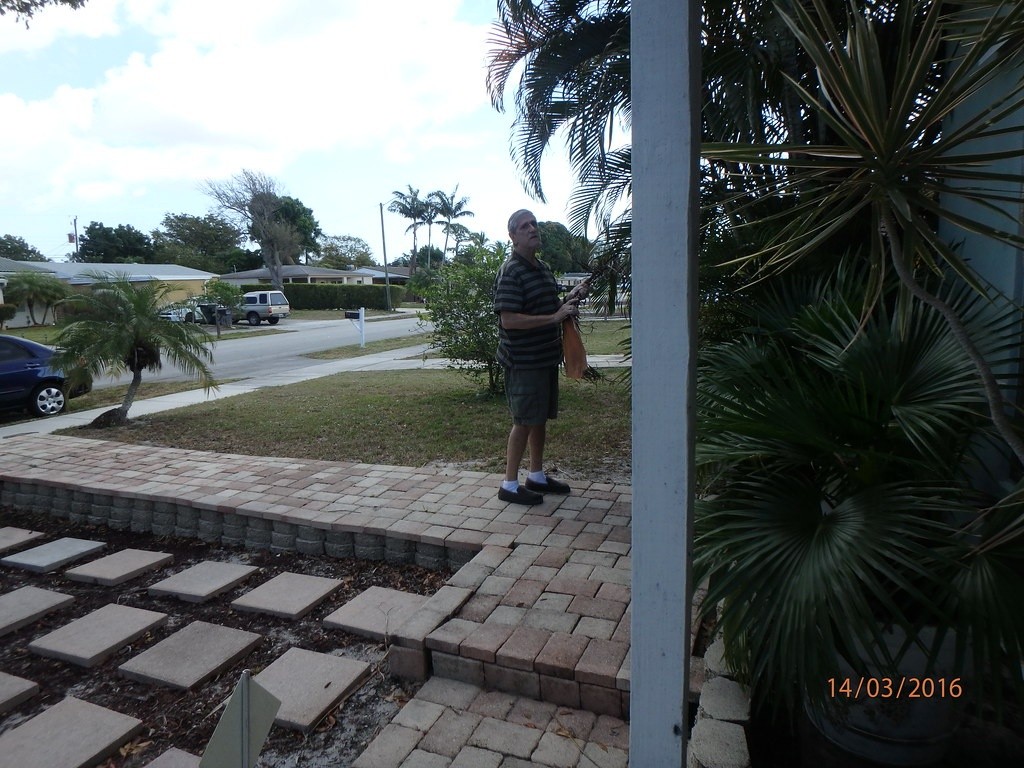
[563,296,567,304]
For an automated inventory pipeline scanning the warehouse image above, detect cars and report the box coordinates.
[158,296,223,323]
[0,334,93,419]
[557,284,567,292]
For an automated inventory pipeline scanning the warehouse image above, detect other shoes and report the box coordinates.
[498,483,543,505]
[525,474,570,492]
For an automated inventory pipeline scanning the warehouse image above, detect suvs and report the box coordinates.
[230,290,291,326]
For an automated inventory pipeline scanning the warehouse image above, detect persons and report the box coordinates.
[493,208,589,505]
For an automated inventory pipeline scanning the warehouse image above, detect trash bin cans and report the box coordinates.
[196,304,219,325]
[218,307,232,326]
